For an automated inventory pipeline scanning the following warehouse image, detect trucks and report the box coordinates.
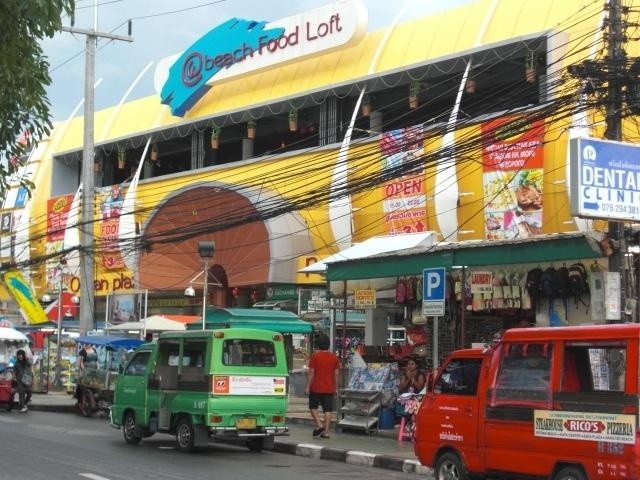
[107,326,291,452]
[412,324,639,479]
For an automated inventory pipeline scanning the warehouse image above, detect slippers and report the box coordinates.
[320,434,329,438]
[313,427,324,436]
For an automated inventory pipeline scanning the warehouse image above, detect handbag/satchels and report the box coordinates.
[400,328,429,359]
[396,277,423,304]
[527,264,589,299]
[362,346,382,363]
[20,373,33,387]
[412,306,428,325]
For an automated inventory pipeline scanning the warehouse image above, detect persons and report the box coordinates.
[79,343,100,373]
[398,358,425,395]
[12,349,34,412]
[304,333,340,439]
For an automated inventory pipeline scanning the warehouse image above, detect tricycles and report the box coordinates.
[74,335,149,417]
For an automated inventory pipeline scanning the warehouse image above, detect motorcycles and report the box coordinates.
[0,363,18,410]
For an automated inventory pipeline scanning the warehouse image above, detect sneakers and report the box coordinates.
[19,404,28,413]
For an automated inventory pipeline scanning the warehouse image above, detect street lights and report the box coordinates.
[197,240,216,330]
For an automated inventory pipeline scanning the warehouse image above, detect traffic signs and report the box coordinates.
[573,138,640,222]
